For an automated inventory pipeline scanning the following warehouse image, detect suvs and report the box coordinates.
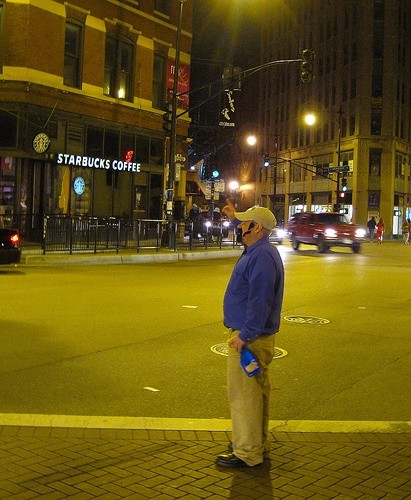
[287,212,364,253]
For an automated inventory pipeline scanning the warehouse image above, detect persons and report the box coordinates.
[188,203,202,239]
[376,217,386,244]
[367,216,376,243]
[401,218,411,246]
[213,197,286,469]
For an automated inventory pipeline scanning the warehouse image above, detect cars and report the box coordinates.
[200,211,230,237]
[0,228,21,264]
[229,218,284,244]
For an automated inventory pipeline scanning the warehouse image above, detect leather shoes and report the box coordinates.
[228,441,233,449]
[216,452,262,467]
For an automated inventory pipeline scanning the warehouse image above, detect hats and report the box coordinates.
[234,206,277,230]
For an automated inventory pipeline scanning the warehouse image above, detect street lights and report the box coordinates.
[305,103,344,212]
[247,129,280,217]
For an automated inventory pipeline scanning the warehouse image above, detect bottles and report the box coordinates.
[240,346,262,377]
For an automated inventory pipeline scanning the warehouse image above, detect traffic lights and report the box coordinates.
[209,163,219,180]
[264,153,270,167]
[342,179,347,191]
